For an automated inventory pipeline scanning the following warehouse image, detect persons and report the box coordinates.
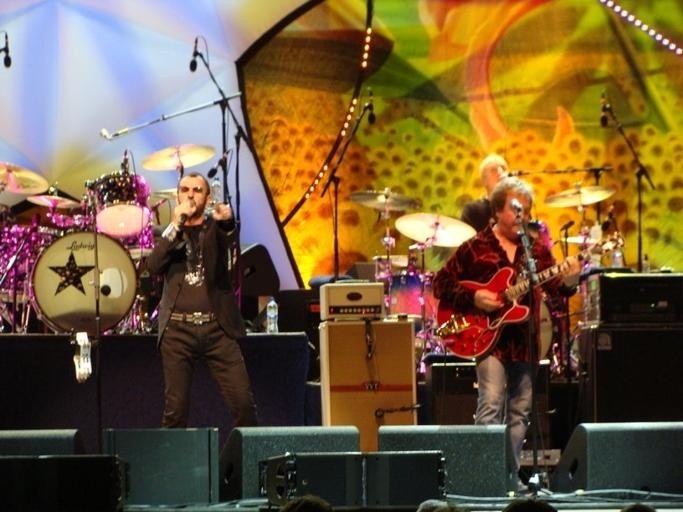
[145,172,260,428]
[458,153,532,231]
[278,494,336,512]
[415,498,458,512]
[431,175,582,499]
[620,502,656,512]
[503,499,558,512]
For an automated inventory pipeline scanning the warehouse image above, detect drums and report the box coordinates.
[29,231,138,335]
[539,299,554,359]
[376,272,440,332]
[0,224,66,304]
[119,220,154,259]
[92,172,151,237]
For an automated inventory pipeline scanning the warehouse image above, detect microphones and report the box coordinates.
[4,35,11,67]
[601,92,609,127]
[601,203,616,232]
[509,199,522,213]
[179,214,188,227]
[189,39,198,72]
[368,88,376,126]
[560,221,574,232]
[207,150,230,178]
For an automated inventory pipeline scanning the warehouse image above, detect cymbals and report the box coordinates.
[26,194,82,210]
[373,255,412,267]
[396,213,477,247]
[545,187,616,209]
[155,188,177,198]
[560,234,599,244]
[349,191,423,212]
[144,144,216,170]
[1,162,49,196]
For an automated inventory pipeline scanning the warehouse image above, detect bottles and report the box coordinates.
[211,176,221,201]
[267,297,278,332]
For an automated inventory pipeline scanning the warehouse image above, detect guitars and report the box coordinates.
[436,230,626,361]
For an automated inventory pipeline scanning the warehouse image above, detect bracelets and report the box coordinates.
[564,283,581,290]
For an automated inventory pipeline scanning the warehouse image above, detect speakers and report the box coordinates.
[229,240,282,329]
[1,453,132,512]
[219,425,361,501]
[549,422,682,497]
[364,450,443,507]
[74,428,221,504]
[377,424,520,499]
[258,451,365,506]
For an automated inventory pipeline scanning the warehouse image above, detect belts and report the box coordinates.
[170,312,217,326]
